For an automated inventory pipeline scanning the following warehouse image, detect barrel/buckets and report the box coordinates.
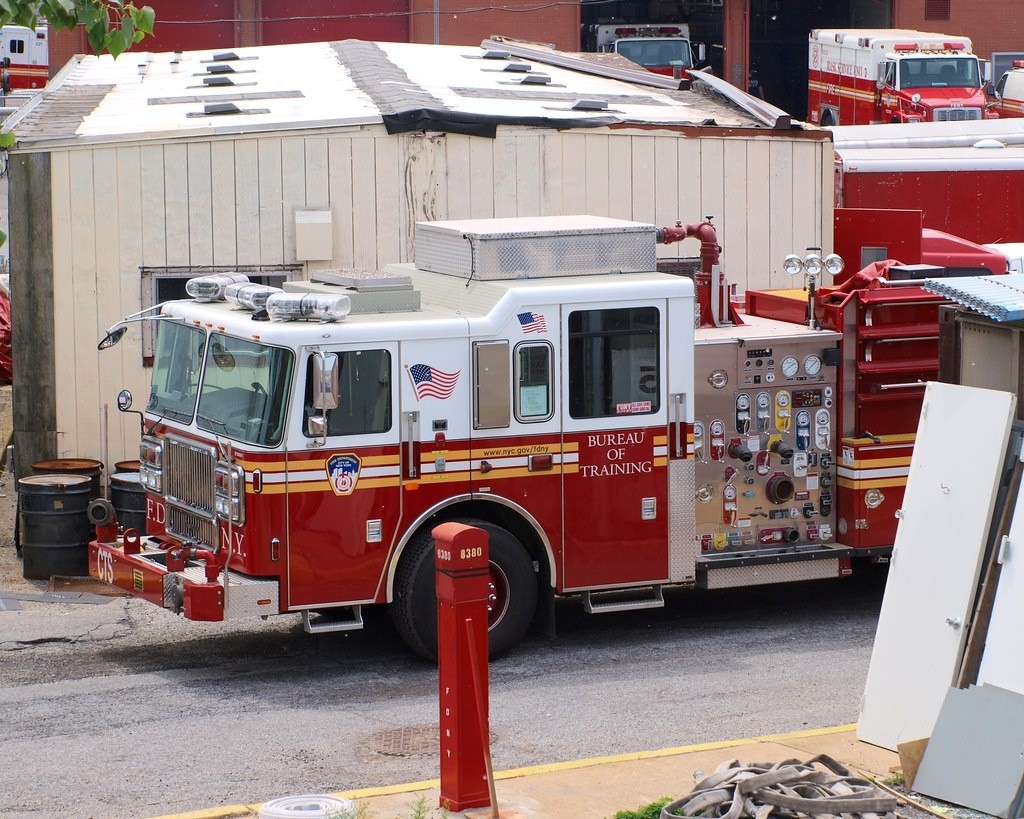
[17,474,93,580]
[29,458,102,540]
[114,460,141,473]
[109,473,146,537]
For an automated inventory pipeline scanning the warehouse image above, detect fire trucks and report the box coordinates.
[86,210,1024,665]
[0,22,48,93]
[580,15,707,82]
[818,118,1024,289]
[979,51,1024,118]
[806,27,1003,126]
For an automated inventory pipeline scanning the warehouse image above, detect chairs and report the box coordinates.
[939,65,955,80]
[329,350,390,435]
[899,62,910,83]
[659,45,673,59]
[620,48,631,59]
